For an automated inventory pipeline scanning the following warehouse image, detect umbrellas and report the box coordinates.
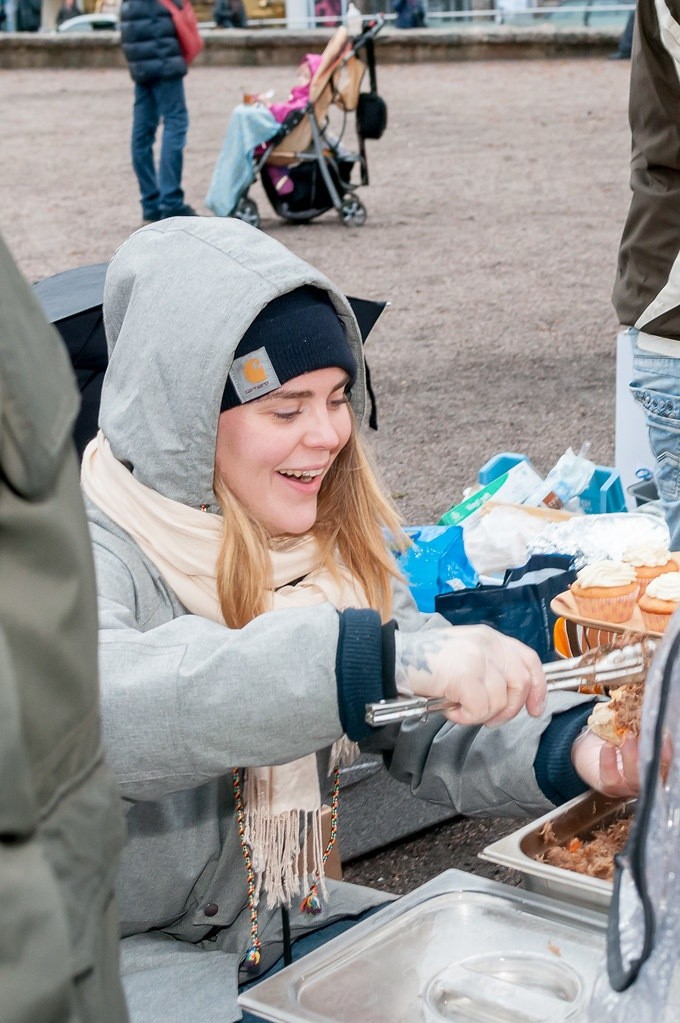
[28,262,388,467]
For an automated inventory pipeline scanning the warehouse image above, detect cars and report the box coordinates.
[47,11,121,36]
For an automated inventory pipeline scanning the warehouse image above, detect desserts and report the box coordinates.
[570,560,639,623]
[621,533,680,603]
[639,571,680,633]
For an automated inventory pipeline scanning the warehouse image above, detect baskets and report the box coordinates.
[481,454,628,516]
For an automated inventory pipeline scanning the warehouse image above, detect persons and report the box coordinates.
[243,54,321,156]
[212,1,540,31]
[78,216,670,1023]
[609,1,679,551]
[0,0,122,34]
[120,0,203,226]
[1,225,129,1023]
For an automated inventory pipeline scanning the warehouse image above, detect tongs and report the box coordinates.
[365,636,658,730]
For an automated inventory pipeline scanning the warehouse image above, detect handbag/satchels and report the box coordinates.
[435,554,577,666]
[355,92,387,139]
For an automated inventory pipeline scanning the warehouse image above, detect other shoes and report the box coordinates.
[607,52,630,59]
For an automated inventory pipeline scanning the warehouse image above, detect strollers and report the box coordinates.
[231,9,389,232]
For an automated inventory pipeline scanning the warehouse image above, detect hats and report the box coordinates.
[221,284,357,418]
